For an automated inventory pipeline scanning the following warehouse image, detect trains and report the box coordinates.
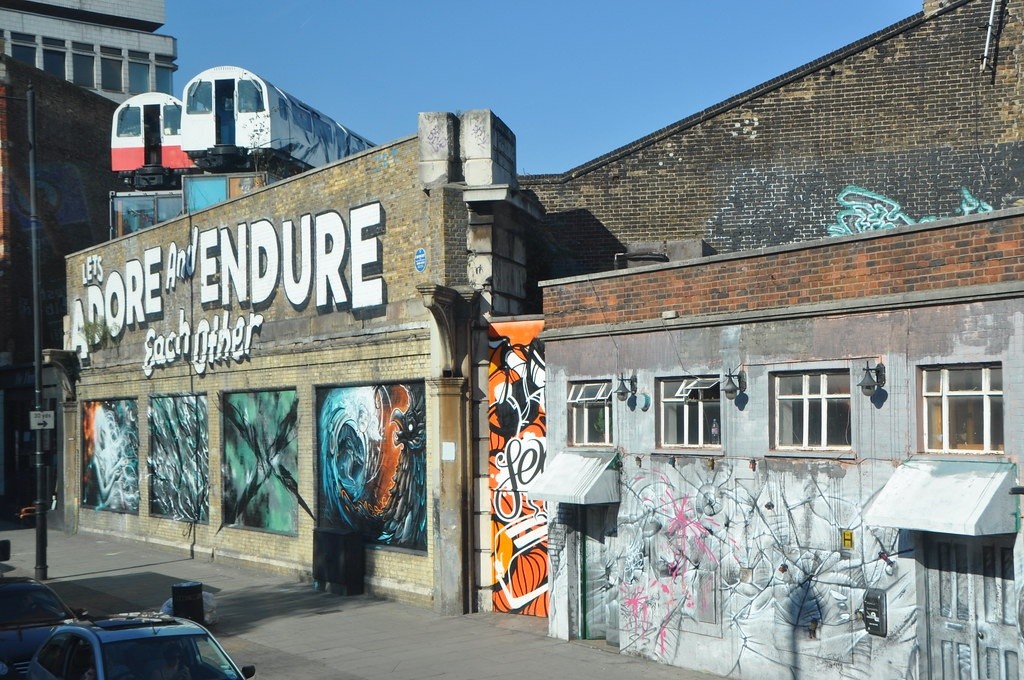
[180,65,384,174]
[109,91,213,241]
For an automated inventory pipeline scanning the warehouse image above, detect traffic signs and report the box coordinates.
[30,410,55,430]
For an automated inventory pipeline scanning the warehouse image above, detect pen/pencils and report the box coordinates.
[312,527,364,596]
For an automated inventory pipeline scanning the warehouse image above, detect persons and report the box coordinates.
[84,648,136,680]
[152,643,190,680]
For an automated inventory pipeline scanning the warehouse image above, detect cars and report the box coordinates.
[0,576,90,680]
[29,611,256,680]
[11,499,58,526]
